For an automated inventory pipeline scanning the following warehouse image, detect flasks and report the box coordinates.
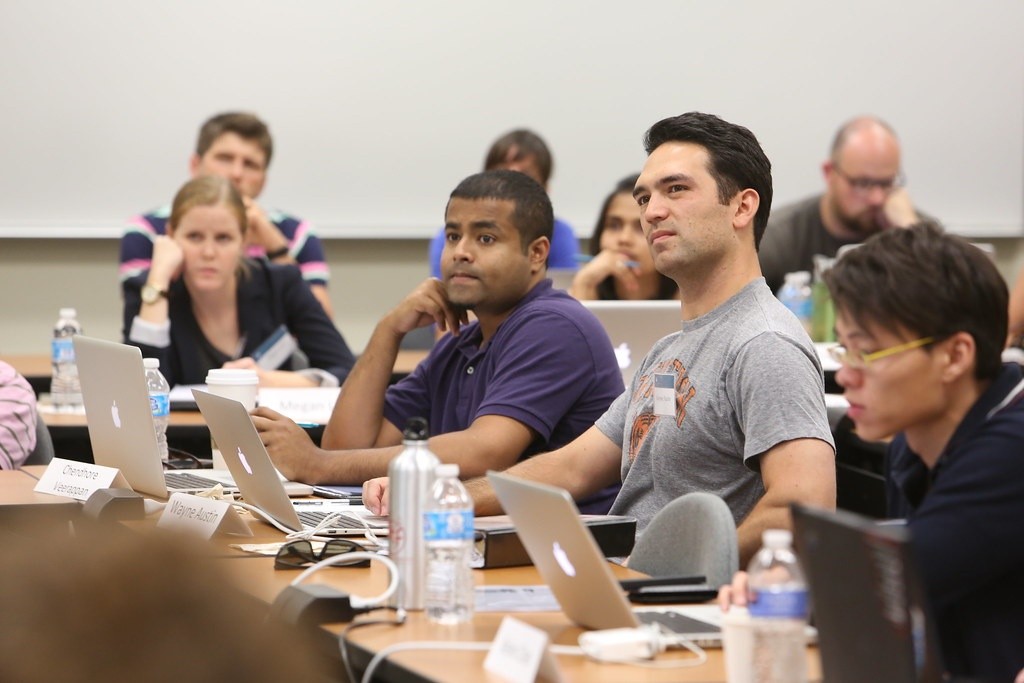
[388,417,443,612]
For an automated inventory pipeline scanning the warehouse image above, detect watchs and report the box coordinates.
[141,284,169,304]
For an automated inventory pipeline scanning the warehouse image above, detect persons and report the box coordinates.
[119,113,332,324]
[717,220,1024,683]
[428,128,582,344]
[0,360,36,471]
[360,112,836,571]
[248,170,625,485]
[756,118,922,298]
[566,173,681,302]
[127,174,356,393]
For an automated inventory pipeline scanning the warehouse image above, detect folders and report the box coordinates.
[474,514,637,569]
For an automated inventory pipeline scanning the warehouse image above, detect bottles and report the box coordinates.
[143,358,170,468]
[779,269,814,338]
[744,529,809,683]
[421,462,473,621]
[49,309,83,411]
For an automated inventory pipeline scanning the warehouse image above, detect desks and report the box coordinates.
[0,349,1024,683]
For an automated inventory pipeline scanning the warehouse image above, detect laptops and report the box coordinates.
[72,334,314,499]
[192,389,392,537]
[483,468,820,646]
[787,493,951,683]
[575,299,684,391]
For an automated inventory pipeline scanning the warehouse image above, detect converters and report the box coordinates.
[578,627,664,660]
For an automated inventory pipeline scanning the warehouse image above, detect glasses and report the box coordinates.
[275,539,371,574]
[828,337,931,367]
[836,163,906,192]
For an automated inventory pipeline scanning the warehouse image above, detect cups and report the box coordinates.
[206,369,259,475]
[721,605,752,683]
